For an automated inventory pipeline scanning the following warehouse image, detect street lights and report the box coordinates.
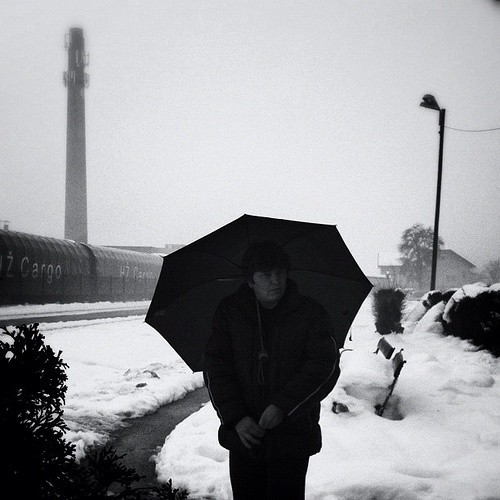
[419,93,448,292]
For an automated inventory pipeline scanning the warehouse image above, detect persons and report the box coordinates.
[202,240,342,500]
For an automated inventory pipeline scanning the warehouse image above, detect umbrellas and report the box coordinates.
[143,213,376,374]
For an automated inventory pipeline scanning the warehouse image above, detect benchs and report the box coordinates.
[331,337,406,417]
